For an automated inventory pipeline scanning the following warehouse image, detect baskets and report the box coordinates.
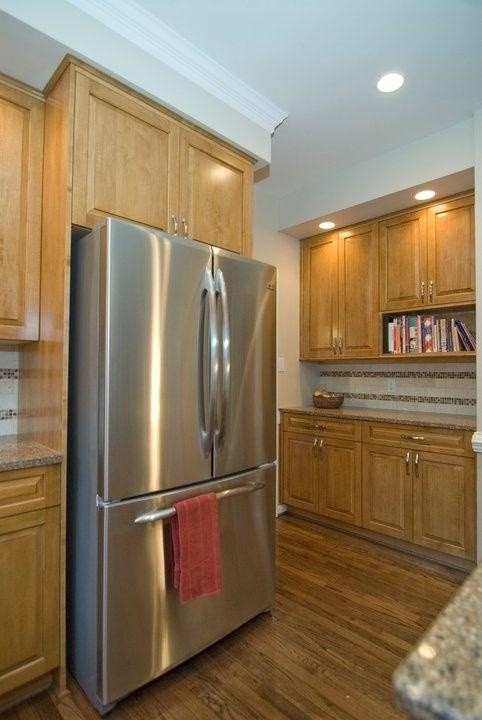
[313,390,343,409]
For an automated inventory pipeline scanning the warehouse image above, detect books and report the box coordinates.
[382,313,477,354]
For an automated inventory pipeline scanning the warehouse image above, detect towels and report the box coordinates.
[170,492,223,604]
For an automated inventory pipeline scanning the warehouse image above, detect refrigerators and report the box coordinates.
[72,217,276,716]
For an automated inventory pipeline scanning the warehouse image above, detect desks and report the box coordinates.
[388,561,482,720]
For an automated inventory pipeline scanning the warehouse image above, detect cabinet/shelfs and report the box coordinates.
[45,55,257,258]
[299,188,475,361]
[1,72,44,347]
[279,411,476,572]
[0,465,68,709]
